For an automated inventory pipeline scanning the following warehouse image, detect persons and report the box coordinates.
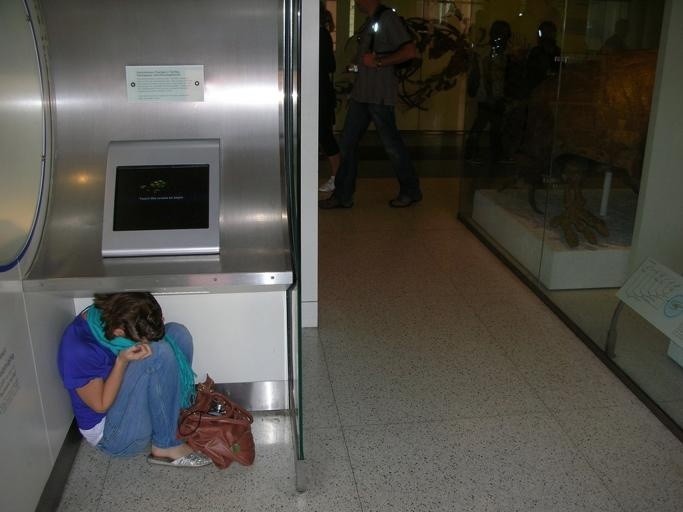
[53,289,221,468]
[318,3,343,194]
[465,19,516,163]
[505,22,564,94]
[319,1,423,210]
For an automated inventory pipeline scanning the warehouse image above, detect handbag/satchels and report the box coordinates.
[176,373,255,470]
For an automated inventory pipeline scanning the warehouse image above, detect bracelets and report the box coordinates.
[375,56,381,68]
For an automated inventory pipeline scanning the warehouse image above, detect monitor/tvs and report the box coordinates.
[101,137,225,264]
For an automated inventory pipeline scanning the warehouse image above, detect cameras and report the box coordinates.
[208,402,224,416]
[345,64,359,73]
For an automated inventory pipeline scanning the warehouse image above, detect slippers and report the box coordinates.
[147,451,212,468]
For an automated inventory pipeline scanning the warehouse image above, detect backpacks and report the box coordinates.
[332,18,374,94]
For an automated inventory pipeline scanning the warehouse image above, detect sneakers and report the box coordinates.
[318,176,336,192]
[318,187,355,208]
[389,189,423,207]
